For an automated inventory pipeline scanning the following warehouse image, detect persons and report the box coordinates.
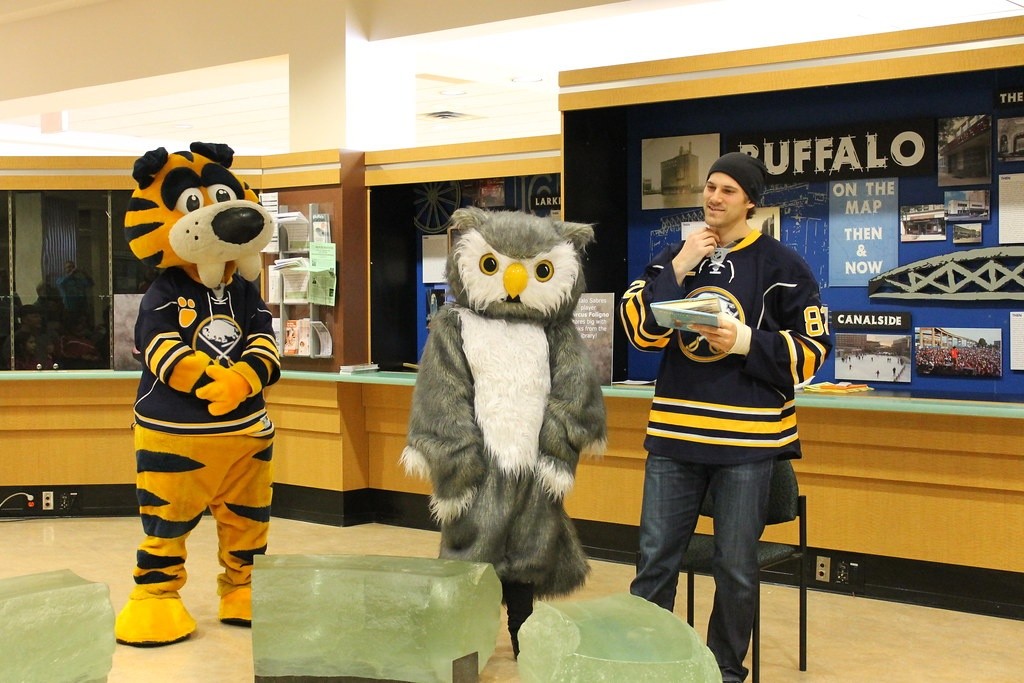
[619,153,833,683]
[841,350,904,381]
[4,262,105,370]
[914,343,1001,377]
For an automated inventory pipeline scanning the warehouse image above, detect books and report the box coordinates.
[650,297,721,334]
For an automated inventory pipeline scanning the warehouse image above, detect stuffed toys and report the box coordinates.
[113,141,281,649]
[401,207,605,660]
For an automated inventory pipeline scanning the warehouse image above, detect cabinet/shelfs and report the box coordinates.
[260,202,335,359]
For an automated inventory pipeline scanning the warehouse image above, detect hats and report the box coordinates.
[707,152,767,206]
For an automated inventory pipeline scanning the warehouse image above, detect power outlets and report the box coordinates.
[23,493,40,515]
[59,492,79,516]
[831,561,848,585]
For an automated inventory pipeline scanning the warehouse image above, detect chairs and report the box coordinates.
[635,495,807,683]
